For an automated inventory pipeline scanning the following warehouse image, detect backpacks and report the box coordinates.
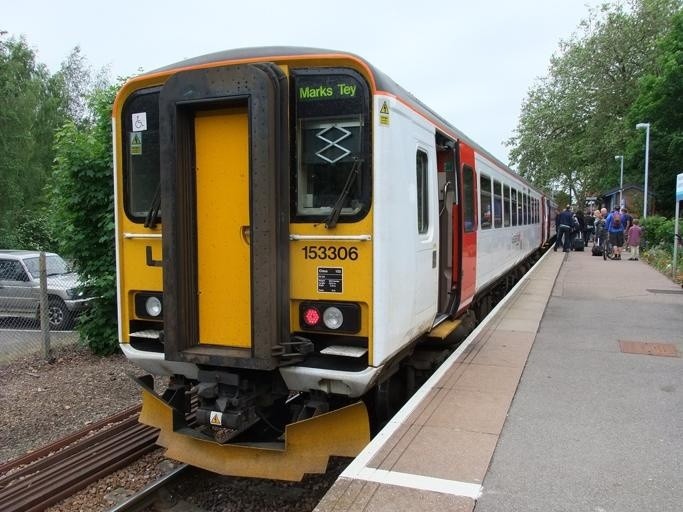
[611,212,621,229]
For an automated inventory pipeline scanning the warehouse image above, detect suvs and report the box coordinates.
[0,249,98,329]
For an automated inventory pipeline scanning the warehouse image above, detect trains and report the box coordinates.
[112,45,558,483]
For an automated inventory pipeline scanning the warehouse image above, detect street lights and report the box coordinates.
[636,123,650,220]
[614,155,624,211]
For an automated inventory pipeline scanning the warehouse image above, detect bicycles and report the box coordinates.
[603,232,613,260]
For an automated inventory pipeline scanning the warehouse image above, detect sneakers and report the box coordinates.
[612,257,622,260]
[554,247,570,252]
[628,258,638,260]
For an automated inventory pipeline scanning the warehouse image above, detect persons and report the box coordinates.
[480,204,537,226]
[552,204,643,260]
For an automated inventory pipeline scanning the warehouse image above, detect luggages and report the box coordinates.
[574,238,584,251]
[591,237,603,256]
